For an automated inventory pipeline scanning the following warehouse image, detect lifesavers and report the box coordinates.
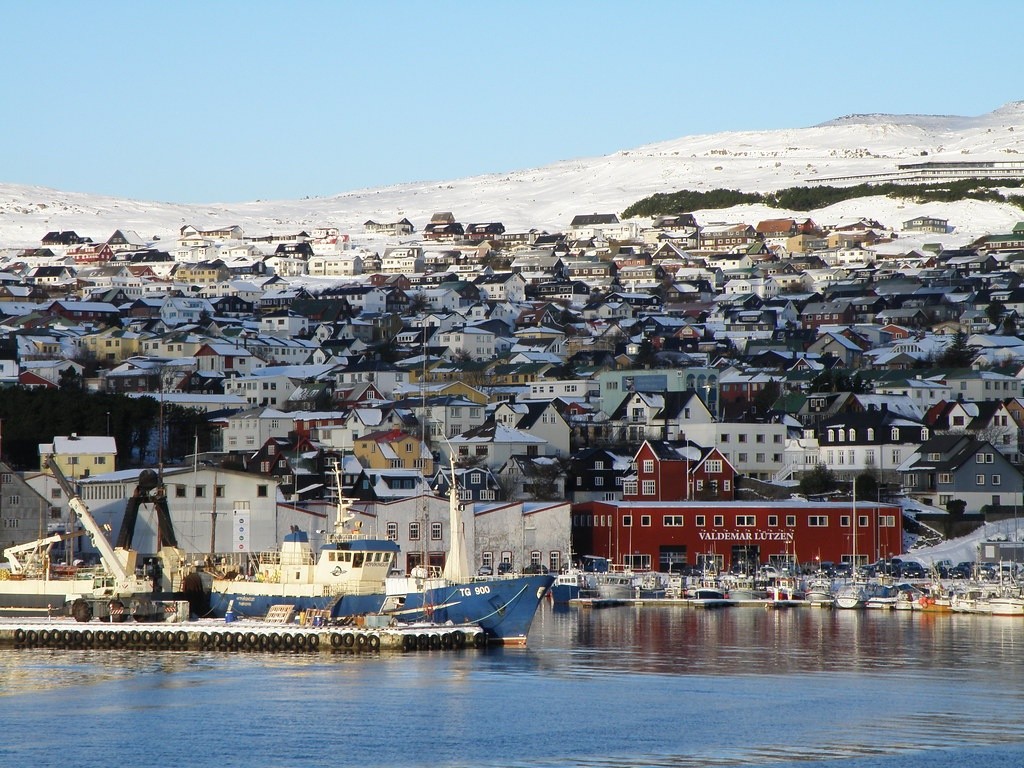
[356,634,368,647]
[308,633,319,647]
[452,631,463,646]
[429,635,440,648]
[473,633,486,648]
[417,634,429,650]
[294,633,306,647]
[14,629,295,647]
[366,635,380,650]
[442,633,452,648]
[402,634,416,649]
[330,633,342,647]
[921,601,930,610]
[342,632,354,647]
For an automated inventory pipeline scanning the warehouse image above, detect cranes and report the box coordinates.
[3,523,112,581]
[40,450,191,623]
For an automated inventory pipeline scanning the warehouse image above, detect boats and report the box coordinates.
[551,475,1024,615]
[209,311,557,647]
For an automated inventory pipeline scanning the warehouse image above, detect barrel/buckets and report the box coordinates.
[314,616,321,625]
[225,612,234,623]
[295,618,300,624]
